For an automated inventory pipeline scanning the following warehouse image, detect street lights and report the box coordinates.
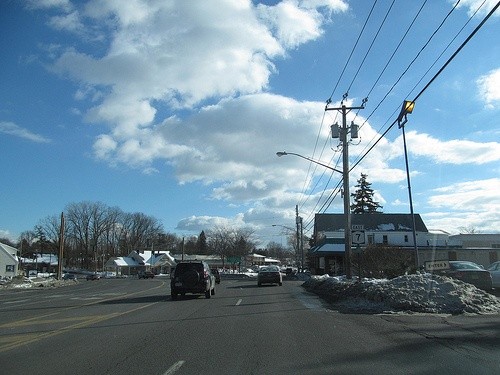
[398,99,421,273]
[272,224,300,274]
[276,151,353,280]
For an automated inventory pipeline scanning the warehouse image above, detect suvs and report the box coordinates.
[170,261,216,299]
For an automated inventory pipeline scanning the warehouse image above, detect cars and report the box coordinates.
[484,260,500,291]
[137,271,154,280]
[257,266,283,286]
[86,274,101,281]
[211,268,221,284]
[430,260,492,294]
[62,274,75,280]
